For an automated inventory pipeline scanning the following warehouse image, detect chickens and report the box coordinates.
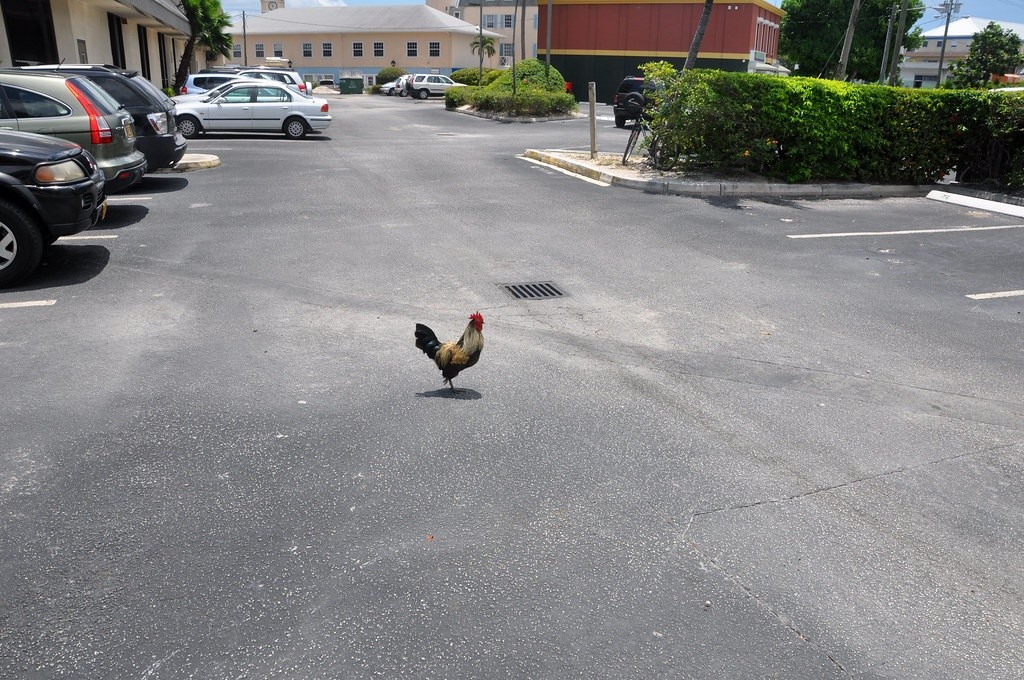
[414,310,485,395]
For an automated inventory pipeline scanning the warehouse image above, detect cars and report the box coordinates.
[379,73,413,97]
[169,63,322,107]
[0,125,108,289]
[172,82,333,139]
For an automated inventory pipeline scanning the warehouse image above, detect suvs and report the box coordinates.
[0,70,149,198]
[613,76,672,128]
[5,63,188,173]
[406,73,469,100]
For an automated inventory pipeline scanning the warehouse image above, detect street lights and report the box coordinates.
[877,5,950,82]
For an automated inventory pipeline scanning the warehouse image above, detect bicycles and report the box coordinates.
[621,99,681,171]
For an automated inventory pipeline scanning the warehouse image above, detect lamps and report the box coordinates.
[391,59,396,67]
[288,60,292,68]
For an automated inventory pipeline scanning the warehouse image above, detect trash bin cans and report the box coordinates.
[340,78,364,94]
[564,82,572,93]
